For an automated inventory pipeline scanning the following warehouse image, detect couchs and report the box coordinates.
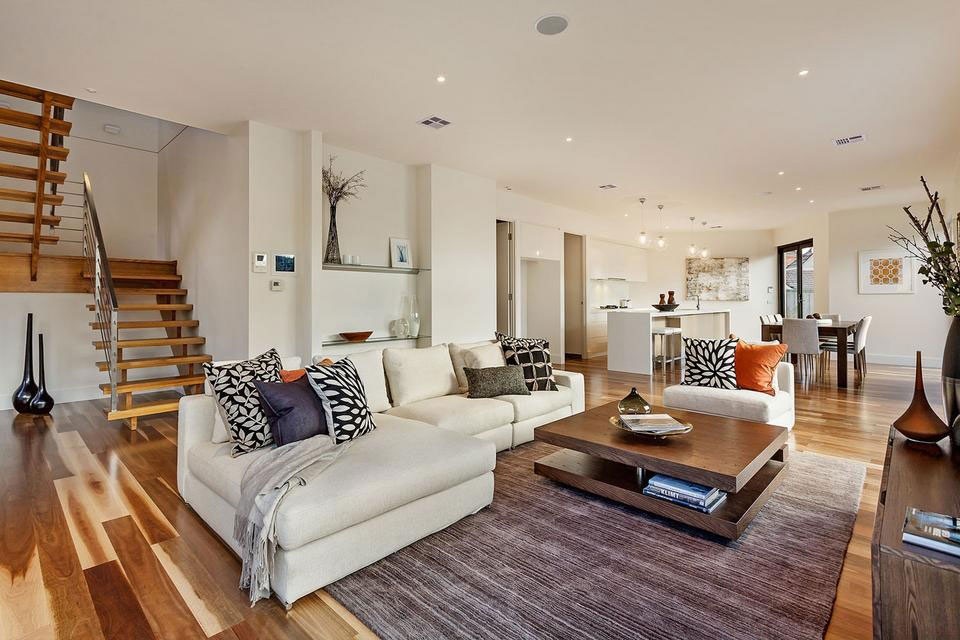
[176,343,590,612]
[663,360,795,437]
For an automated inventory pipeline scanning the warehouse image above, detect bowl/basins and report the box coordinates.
[339,330,373,342]
[652,304,679,312]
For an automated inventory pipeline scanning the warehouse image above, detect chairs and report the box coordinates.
[759,314,825,380]
[781,318,826,394]
[818,315,873,387]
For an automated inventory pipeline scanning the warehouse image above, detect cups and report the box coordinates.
[392,318,408,338]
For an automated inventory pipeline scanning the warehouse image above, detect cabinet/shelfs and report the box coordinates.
[319,263,433,350]
[871,426,960,640]
[588,277,652,312]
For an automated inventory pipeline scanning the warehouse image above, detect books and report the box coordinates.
[648,473,716,498]
[642,484,728,513]
[620,413,685,431]
[901,505,960,557]
[645,483,720,507]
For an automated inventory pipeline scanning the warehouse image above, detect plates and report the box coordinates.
[608,413,693,439]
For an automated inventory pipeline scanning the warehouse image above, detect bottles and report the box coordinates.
[408,295,420,337]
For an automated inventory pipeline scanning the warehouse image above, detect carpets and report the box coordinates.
[322,441,866,640]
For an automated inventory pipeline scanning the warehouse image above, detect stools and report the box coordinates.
[651,326,685,377]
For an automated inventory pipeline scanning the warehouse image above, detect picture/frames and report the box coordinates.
[858,247,915,293]
[388,237,413,268]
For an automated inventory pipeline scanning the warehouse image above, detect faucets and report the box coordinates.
[691,294,703,310]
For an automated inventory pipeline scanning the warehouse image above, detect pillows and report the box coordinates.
[461,341,508,367]
[682,336,740,390]
[251,374,329,448]
[500,342,558,392]
[495,330,548,342]
[206,354,302,445]
[312,349,393,413]
[448,340,506,392]
[305,352,378,445]
[729,333,788,397]
[743,338,782,392]
[203,345,287,459]
[382,346,464,407]
[462,366,533,398]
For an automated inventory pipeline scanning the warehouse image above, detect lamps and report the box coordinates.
[638,196,708,258]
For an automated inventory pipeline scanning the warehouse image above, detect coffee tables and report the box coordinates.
[533,396,791,540]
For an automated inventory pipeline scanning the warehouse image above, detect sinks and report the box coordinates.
[675,308,697,312]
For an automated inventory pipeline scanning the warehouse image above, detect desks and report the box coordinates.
[760,321,857,390]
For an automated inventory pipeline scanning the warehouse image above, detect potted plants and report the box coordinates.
[885,171,960,441]
